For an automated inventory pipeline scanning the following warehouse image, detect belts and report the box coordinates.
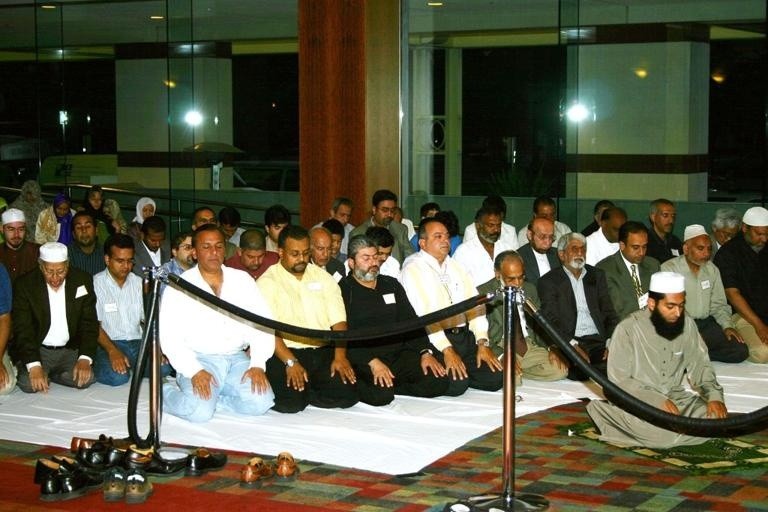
[41,344,66,350]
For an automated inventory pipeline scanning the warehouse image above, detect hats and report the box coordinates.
[742,205,768,226]
[1,206,26,225]
[39,241,68,263]
[649,271,686,295]
[683,223,709,243]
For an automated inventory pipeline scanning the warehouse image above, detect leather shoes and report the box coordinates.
[32,432,299,505]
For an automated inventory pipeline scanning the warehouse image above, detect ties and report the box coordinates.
[630,265,642,298]
